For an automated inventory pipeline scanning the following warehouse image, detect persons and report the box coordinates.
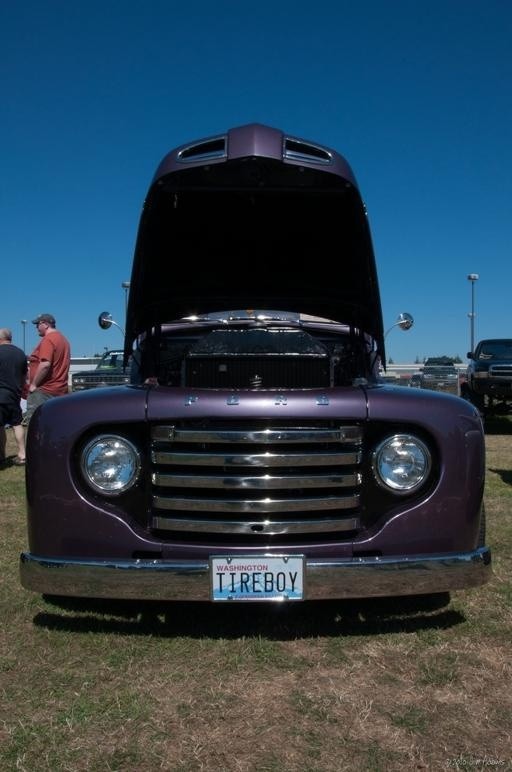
[1,328,27,472]
[21,314,71,448]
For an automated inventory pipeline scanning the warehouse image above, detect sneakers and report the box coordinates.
[7,454,27,466]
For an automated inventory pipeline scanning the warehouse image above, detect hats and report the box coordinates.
[32,313,55,324]
